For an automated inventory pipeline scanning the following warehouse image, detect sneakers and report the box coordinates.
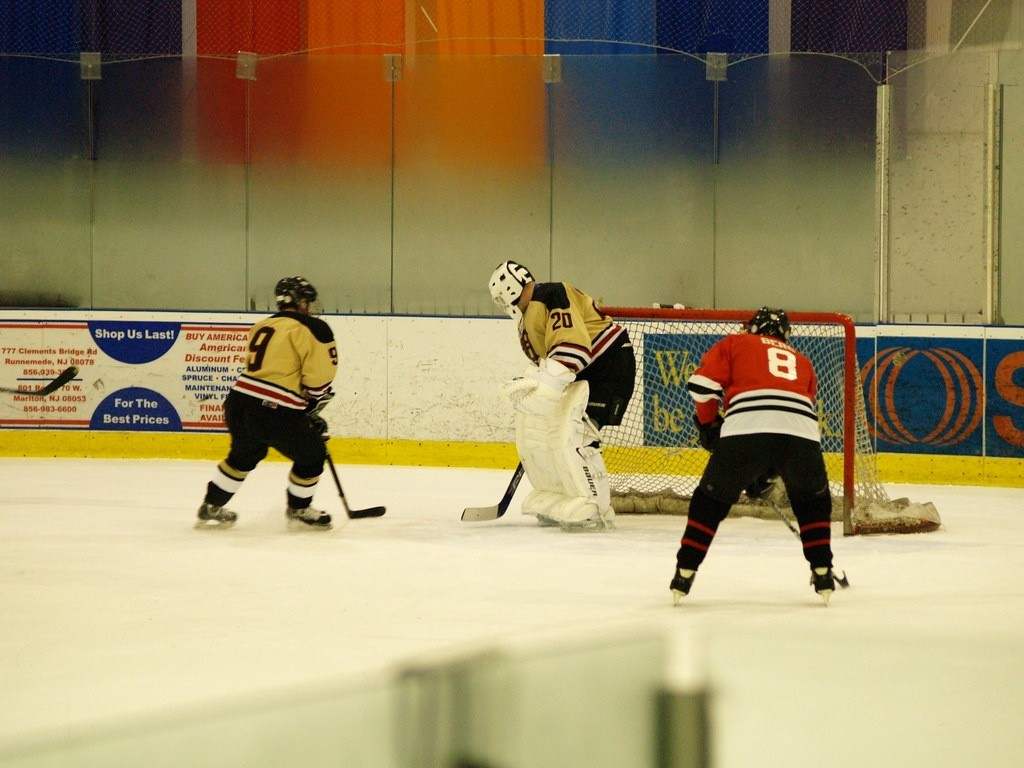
[286,506,333,532]
[809,567,836,607]
[192,501,237,530]
[669,567,697,607]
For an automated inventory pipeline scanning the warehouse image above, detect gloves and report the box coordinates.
[692,414,724,453]
[744,475,775,501]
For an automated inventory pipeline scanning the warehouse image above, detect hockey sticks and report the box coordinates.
[460,457,531,522]
[325,448,387,521]
[764,497,853,587]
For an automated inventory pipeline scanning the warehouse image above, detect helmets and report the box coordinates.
[488,260,536,325]
[274,276,317,305]
[748,306,791,342]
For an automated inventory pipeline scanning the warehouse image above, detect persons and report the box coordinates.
[670,306,835,608]
[192,276,338,532]
[488,260,636,533]
